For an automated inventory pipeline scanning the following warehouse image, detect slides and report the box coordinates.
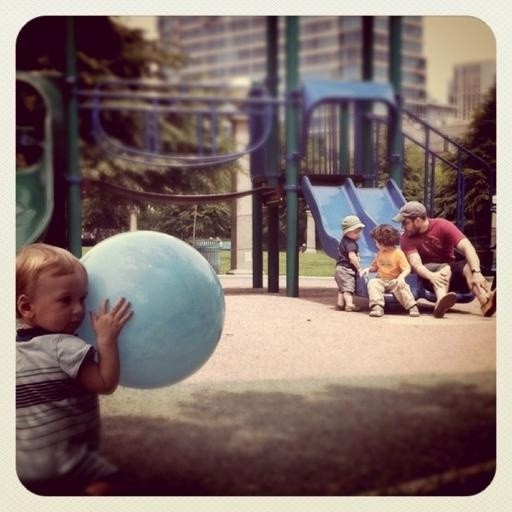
[16,166,55,248]
[301,175,475,303]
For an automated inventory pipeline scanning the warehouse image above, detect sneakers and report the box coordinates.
[434,292,456,317]
[337,304,420,316]
[481,289,496,317]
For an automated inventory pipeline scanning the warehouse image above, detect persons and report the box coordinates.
[16,242,137,495]
[334,215,366,312]
[360,223,421,317]
[392,200,496,318]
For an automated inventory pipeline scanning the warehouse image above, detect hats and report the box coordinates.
[341,216,366,235]
[393,201,427,222]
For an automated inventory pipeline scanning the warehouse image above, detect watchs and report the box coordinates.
[472,267,482,273]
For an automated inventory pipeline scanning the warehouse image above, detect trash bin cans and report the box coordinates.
[195,238,219,276]
